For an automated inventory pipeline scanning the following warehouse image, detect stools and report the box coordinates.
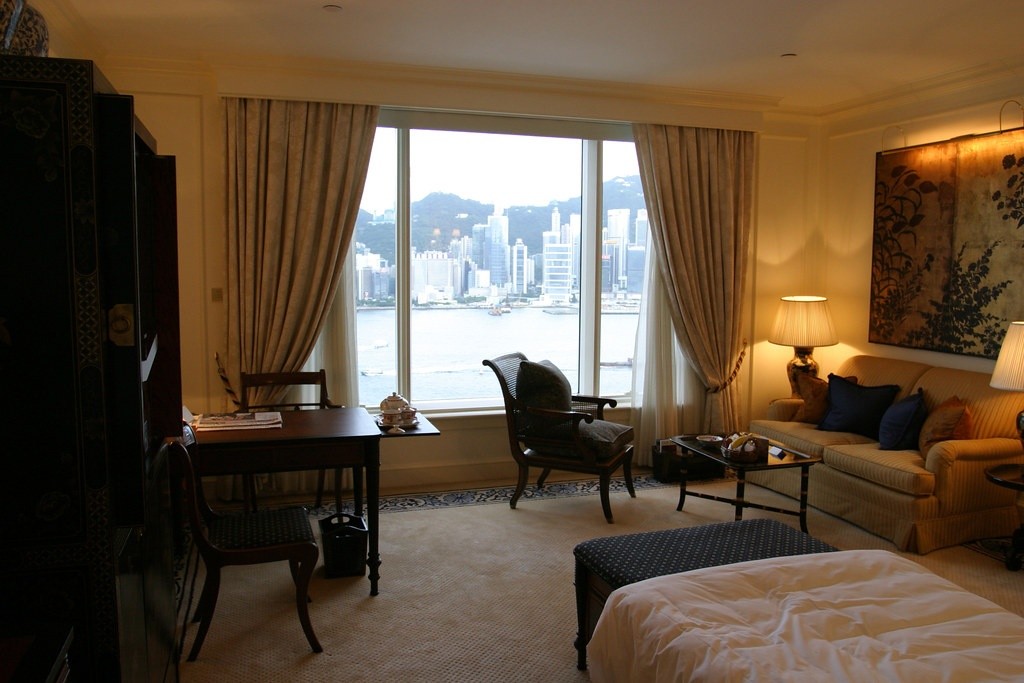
[573,519,1024,683]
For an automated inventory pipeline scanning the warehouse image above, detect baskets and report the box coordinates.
[720,431,758,461]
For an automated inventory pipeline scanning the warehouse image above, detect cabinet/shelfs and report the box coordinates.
[0,54,183,683]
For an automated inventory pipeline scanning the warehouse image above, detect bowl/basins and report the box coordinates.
[696,435,724,449]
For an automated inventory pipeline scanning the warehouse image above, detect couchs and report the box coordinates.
[744,354,1024,556]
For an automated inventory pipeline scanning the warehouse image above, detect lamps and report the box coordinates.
[989,320,1024,446]
[768,295,840,397]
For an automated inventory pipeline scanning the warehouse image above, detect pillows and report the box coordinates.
[790,371,858,424]
[919,394,973,461]
[878,386,927,451]
[815,373,901,442]
[515,361,572,419]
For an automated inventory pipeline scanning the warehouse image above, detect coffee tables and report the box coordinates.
[670,433,822,535]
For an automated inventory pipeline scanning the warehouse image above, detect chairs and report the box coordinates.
[239,369,344,511]
[483,353,636,524]
[169,421,323,662]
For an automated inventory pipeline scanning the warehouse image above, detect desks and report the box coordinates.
[194,408,441,596]
[983,464,1024,573]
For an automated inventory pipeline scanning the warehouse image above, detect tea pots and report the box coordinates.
[379,392,410,423]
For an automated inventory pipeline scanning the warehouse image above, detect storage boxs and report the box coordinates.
[652,444,725,481]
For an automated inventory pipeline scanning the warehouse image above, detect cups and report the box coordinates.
[398,403,416,424]
[382,409,401,424]
